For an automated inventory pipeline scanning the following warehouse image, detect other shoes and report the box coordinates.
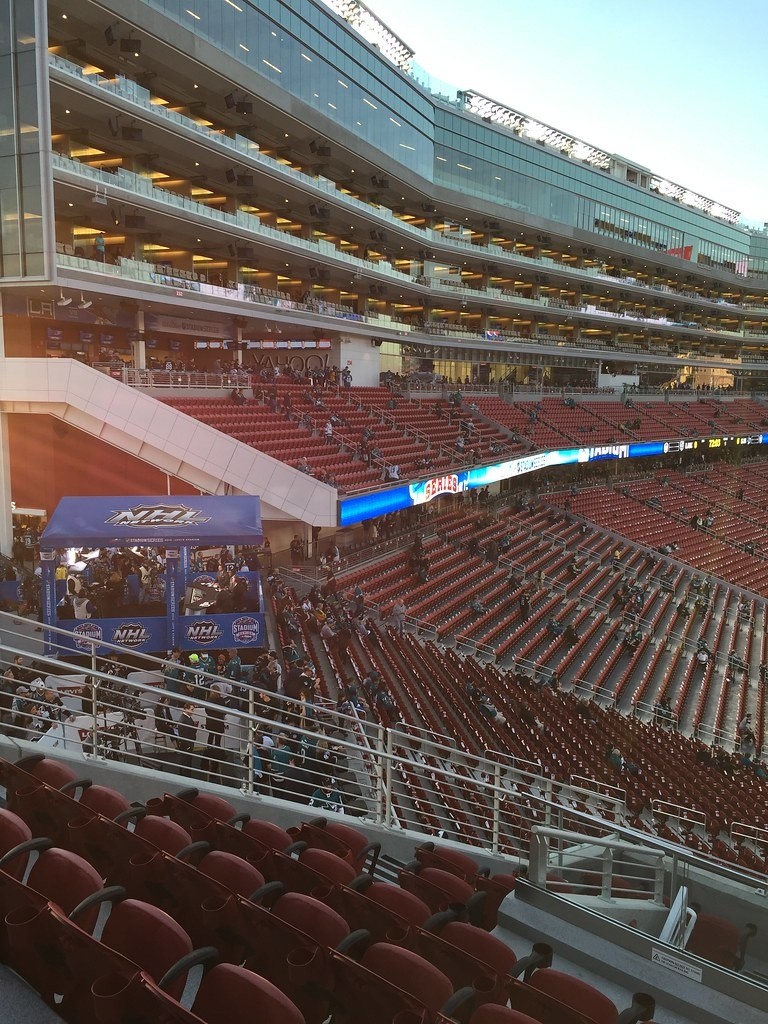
[175,747,181,755]
[34,628,42,632]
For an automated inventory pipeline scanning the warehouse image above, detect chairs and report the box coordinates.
[0,346,768,1024]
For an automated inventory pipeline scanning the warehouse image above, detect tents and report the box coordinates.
[38,495,264,655]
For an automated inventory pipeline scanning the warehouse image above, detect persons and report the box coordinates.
[115,247,124,265]
[94,232,105,262]
[0,346,768,814]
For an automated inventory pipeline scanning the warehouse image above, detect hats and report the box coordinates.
[268,651,279,660]
[612,748,620,756]
[280,732,290,745]
[322,777,335,787]
[189,654,198,663]
[16,686,30,694]
